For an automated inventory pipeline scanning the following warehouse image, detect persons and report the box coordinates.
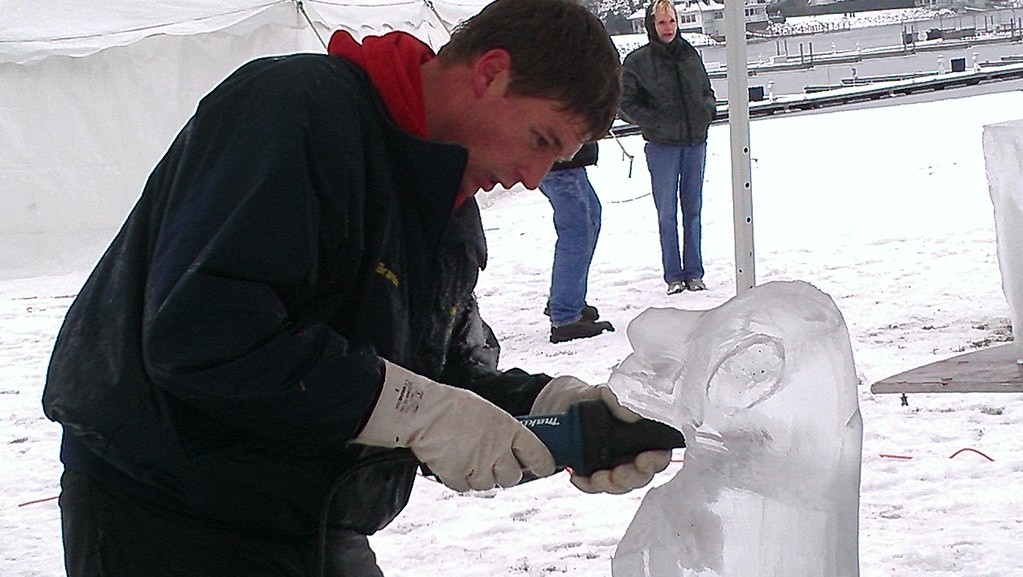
[537,139,616,343]
[42,0,675,577]
[617,0,717,297]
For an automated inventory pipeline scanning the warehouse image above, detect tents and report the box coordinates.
[0,0,483,275]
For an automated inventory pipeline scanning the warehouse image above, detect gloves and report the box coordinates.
[349,356,554,492]
[529,374,673,494]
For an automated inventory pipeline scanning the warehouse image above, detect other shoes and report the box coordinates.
[685,279,707,292]
[550,317,614,344]
[668,281,684,295]
[544,301,599,321]
